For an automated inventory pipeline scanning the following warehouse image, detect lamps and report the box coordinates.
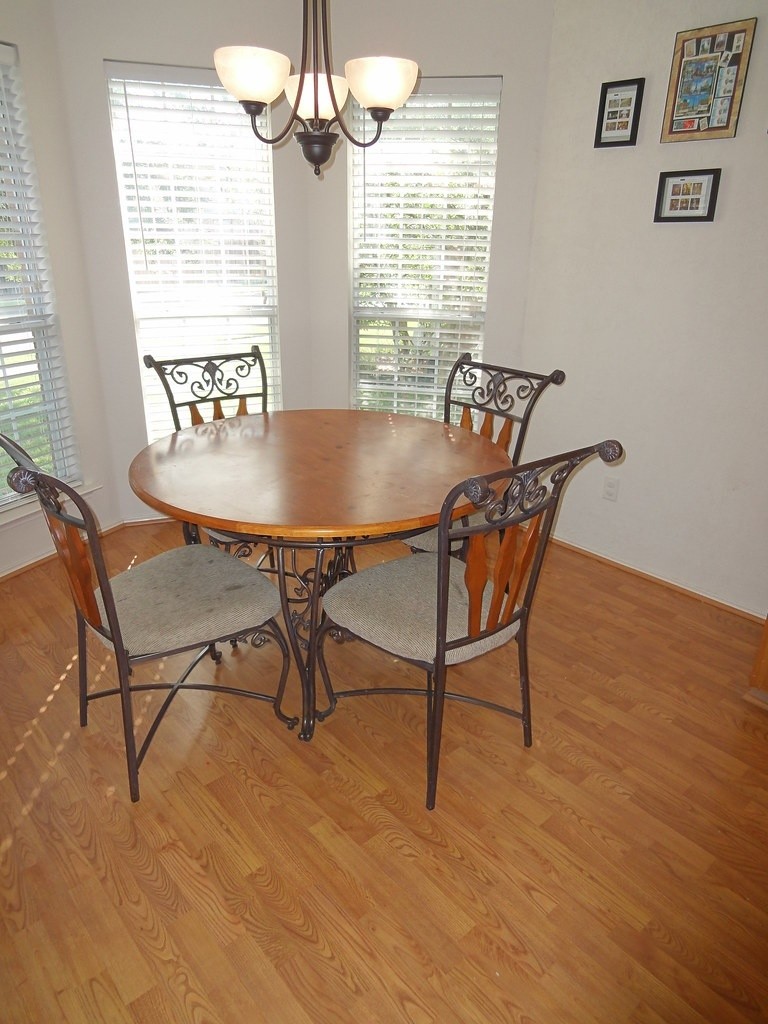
[214,0,418,176]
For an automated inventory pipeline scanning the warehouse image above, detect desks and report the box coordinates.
[129,409,513,741]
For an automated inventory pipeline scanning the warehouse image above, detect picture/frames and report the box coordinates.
[653,168,722,221]
[659,17,757,143]
[593,77,645,148]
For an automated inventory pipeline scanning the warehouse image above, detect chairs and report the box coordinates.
[0,433,300,803]
[303,440,623,810]
[143,345,275,644]
[401,352,565,594]
